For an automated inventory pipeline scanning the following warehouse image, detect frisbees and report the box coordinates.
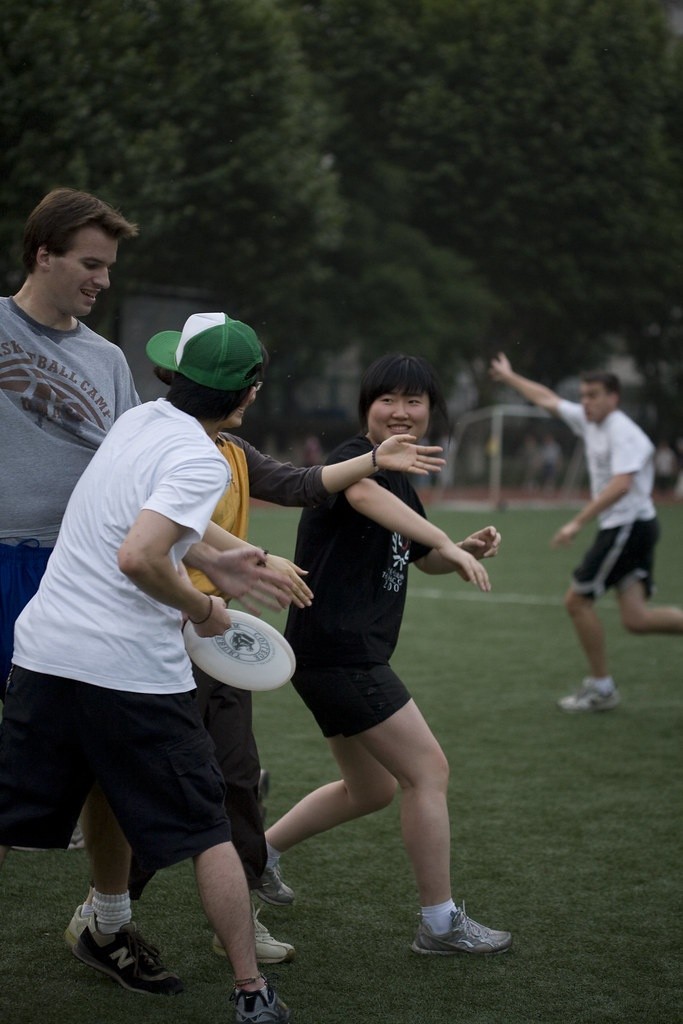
[182,607,297,692]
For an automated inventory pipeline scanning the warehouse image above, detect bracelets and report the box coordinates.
[186,593,213,625]
[370,442,383,471]
[256,550,269,569]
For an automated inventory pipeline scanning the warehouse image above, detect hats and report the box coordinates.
[145,313,261,391]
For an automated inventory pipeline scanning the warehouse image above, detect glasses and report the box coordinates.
[254,381,262,391]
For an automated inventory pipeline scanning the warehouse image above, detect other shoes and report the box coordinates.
[11,826,84,851]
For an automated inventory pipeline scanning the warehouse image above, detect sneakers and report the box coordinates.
[412,907,512,956]
[254,865,295,906]
[73,915,183,995]
[63,905,136,946]
[234,978,289,1024]
[214,919,296,963]
[558,686,621,713]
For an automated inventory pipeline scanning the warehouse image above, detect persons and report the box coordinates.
[253,350,512,959]
[489,350,682,714]
[0,307,305,1024]
[59,365,447,966]
[0,183,137,853]
